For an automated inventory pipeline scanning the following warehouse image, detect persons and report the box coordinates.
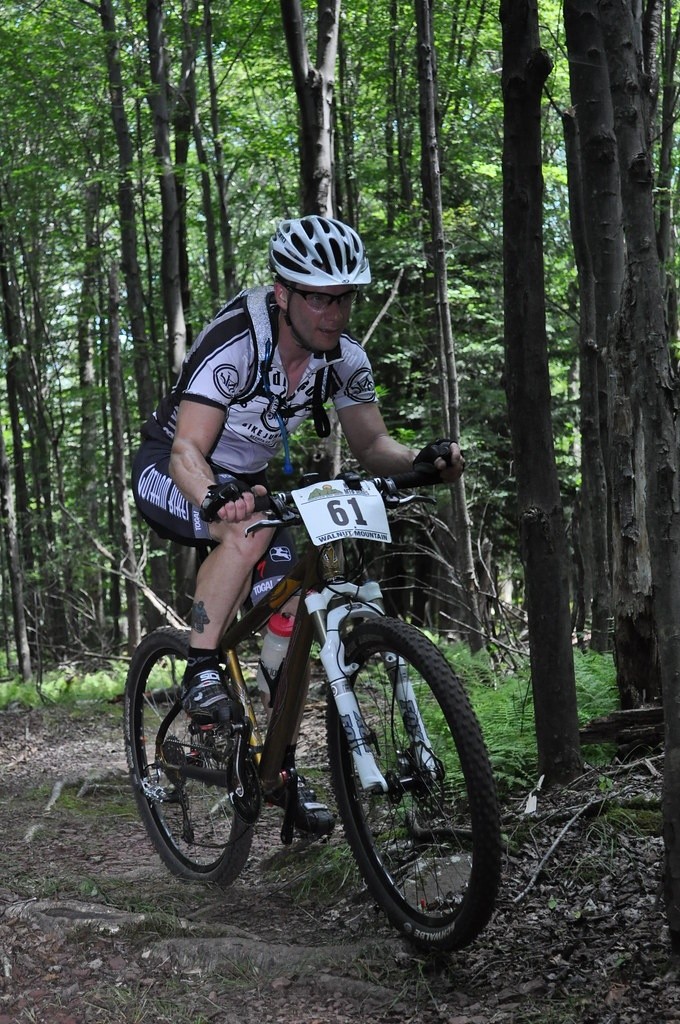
[132,215,467,832]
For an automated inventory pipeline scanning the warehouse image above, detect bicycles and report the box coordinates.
[121,452,502,955]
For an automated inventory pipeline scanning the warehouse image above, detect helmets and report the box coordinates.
[268,214,371,286]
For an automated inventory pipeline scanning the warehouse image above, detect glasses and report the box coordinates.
[274,277,360,309]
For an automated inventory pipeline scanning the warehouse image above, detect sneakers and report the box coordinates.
[263,775,335,834]
[180,671,245,725]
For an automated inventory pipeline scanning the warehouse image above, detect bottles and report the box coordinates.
[256,613,295,694]
